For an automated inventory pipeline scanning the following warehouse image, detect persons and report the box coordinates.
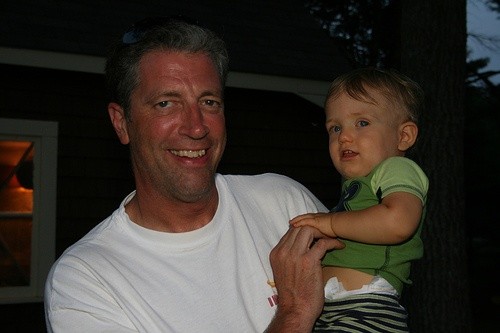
[44,22,344,333]
[288,64,430,333]
